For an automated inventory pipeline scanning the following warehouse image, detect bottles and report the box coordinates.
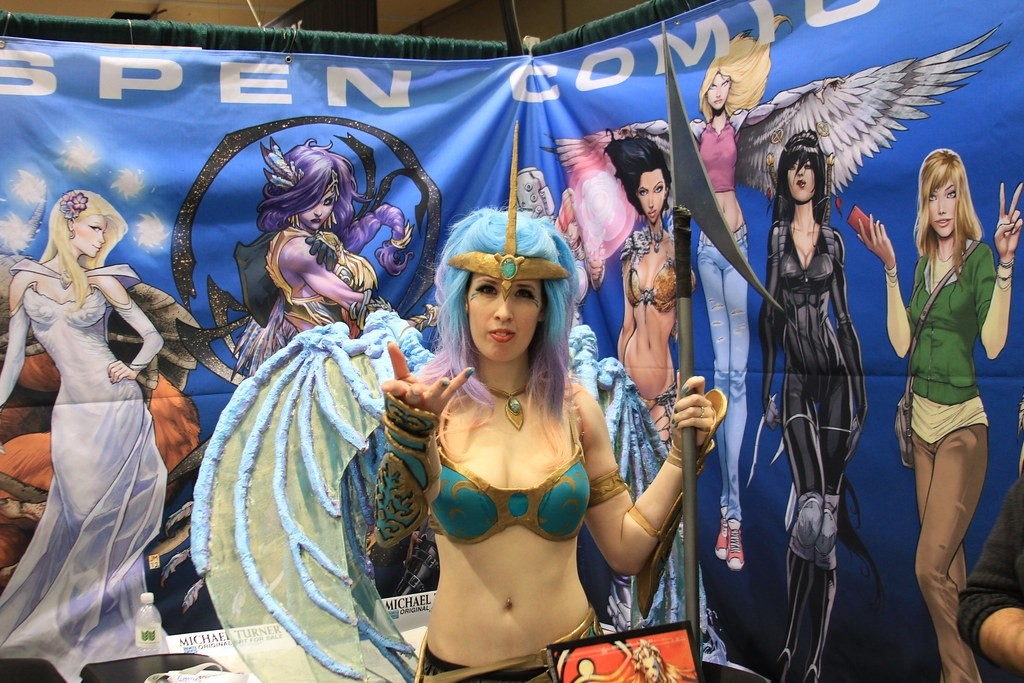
[134,593,161,655]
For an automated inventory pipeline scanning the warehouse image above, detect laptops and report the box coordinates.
[547,620,705,683]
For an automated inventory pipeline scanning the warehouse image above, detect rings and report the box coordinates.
[699,406,705,418]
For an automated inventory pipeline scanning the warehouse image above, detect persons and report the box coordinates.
[956,471,1024,677]
[373,206,716,682]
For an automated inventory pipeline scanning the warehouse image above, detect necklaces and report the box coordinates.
[486,383,526,431]
